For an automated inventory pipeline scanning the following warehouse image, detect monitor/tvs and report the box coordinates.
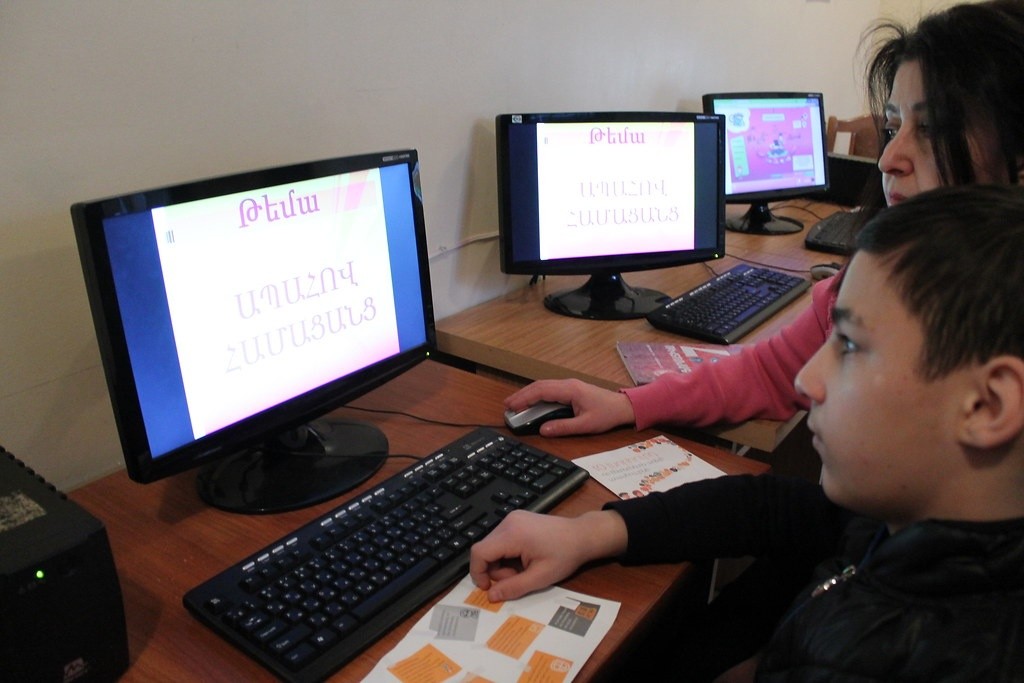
[702,92,830,234]
[68,147,439,516]
[496,111,726,319]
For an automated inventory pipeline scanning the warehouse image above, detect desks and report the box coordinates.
[64,360,775,683]
[430,199,856,459]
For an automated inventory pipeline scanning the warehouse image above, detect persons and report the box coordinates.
[469,185,1023,683]
[502,0,1023,436]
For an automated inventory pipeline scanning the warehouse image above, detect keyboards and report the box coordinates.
[182,428,589,683]
[645,264,812,345]
[806,210,856,254]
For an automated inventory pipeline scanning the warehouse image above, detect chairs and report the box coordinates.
[824,112,888,161]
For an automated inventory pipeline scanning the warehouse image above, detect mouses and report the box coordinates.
[503,399,573,436]
[810,262,844,281]
